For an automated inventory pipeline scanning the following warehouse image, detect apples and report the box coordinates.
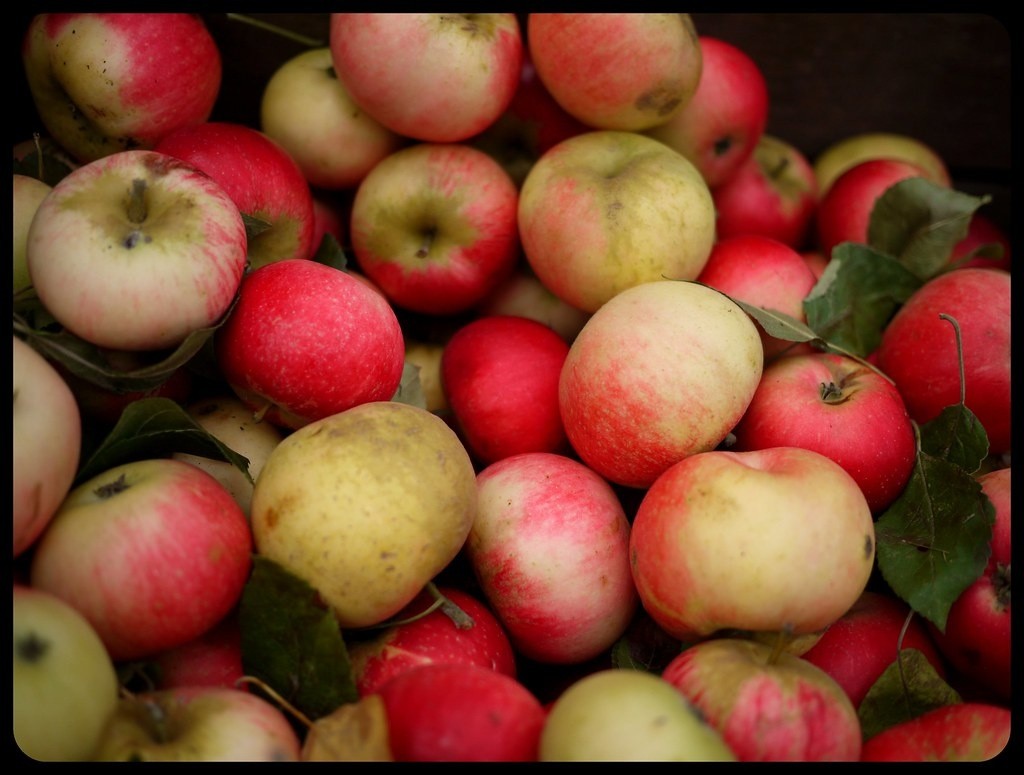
[12,13,1011,760]
